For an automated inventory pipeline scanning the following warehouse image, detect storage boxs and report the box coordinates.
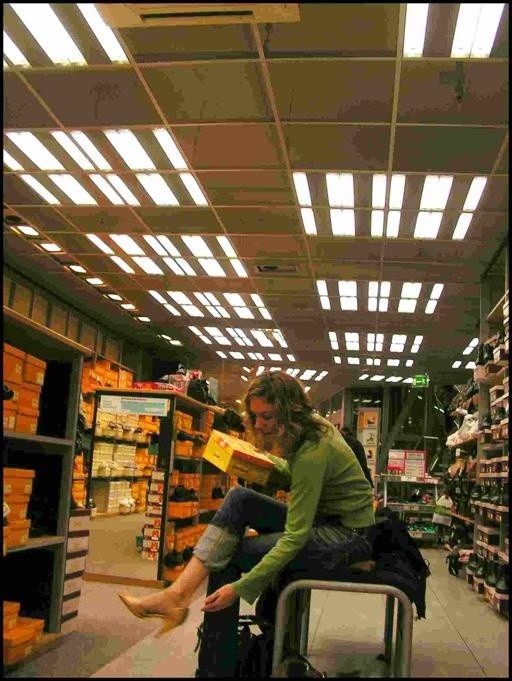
[202,430,274,486]
[388,449,426,476]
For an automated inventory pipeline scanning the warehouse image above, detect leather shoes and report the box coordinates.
[470,485,480,500]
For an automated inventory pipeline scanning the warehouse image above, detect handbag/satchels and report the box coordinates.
[194,614,290,679]
[431,495,454,527]
[454,492,469,514]
[187,380,209,401]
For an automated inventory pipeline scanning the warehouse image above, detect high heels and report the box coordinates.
[117,589,190,639]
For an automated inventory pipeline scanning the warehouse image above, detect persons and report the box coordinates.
[117,372,375,678]
[339,427,374,487]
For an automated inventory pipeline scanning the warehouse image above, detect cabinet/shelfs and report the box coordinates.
[447,291,510,612]
[384,474,439,548]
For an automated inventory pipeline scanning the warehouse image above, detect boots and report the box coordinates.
[496,566,509,594]
[485,560,497,586]
[475,345,484,365]
[483,344,493,359]
[488,488,500,504]
[475,559,486,578]
[469,553,478,570]
[449,555,459,576]
[494,407,505,424]
[480,486,490,502]
[482,413,492,427]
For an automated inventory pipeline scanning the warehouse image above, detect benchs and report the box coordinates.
[272,580,413,679]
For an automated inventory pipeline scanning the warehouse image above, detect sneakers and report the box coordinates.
[198,436,207,443]
[213,488,224,498]
[184,549,193,560]
[177,433,195,440]
[166,552,184,567]
[185,491,198,501]
[174,485,185,502]
